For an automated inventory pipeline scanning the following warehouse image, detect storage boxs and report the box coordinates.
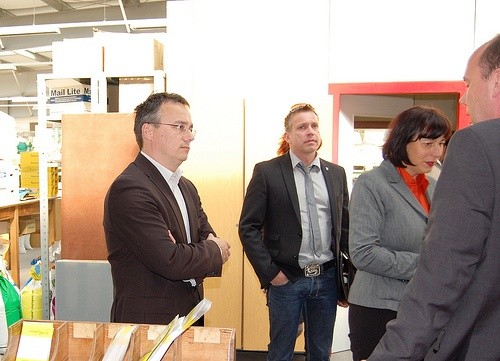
[1,318,236,361]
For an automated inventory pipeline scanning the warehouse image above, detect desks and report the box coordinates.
[0,196,61,289]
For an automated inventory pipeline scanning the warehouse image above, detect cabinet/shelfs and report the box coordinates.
[61,95,334,353]
